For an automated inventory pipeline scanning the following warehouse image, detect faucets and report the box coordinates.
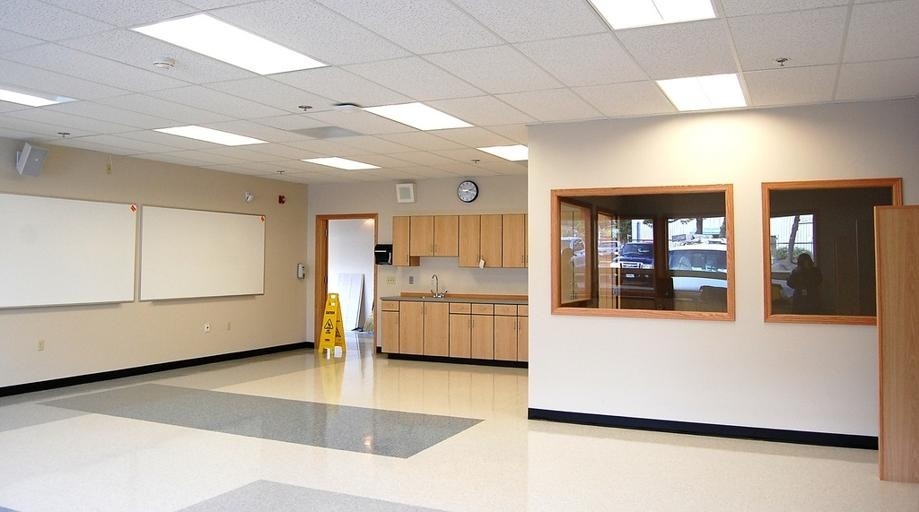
[431,274,438,298]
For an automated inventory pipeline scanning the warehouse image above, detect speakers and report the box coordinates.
[16,142,48,176]
[396,183,417,203]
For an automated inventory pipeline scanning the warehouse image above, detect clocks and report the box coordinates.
[456,180,478,203]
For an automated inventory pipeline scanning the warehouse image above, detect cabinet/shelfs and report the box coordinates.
[393,212,528,269]
[381,300,529,368]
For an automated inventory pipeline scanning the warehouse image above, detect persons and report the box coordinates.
[787,253,824,316]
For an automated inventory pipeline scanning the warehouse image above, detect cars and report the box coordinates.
[561,237,795,305]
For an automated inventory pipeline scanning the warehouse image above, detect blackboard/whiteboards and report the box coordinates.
[0,191,140,310]
[138,204,266,302]
